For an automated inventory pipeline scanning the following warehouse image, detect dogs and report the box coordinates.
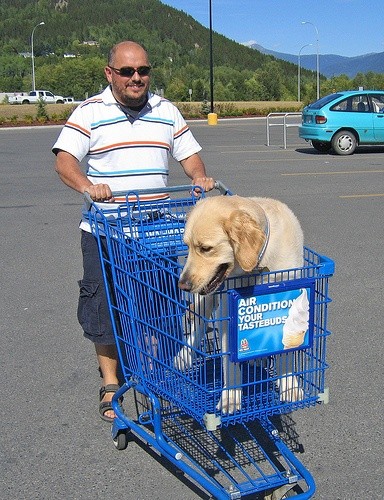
[174,194,304,414]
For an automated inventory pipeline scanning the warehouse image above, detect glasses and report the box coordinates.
[110,64,153,75]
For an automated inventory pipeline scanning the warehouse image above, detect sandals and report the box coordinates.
[99,383,124,422]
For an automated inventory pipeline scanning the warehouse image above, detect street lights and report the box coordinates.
[299,21,319,101]
[298,43,313,101]
[32,22,45,90]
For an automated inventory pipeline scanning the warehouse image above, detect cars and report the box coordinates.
[298,90,384,155]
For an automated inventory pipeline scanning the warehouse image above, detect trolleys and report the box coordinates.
[82,179,336,500]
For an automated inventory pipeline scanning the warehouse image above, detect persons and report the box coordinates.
[51,41,214,422]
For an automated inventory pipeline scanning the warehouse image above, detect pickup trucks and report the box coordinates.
[15,90,65,104]
